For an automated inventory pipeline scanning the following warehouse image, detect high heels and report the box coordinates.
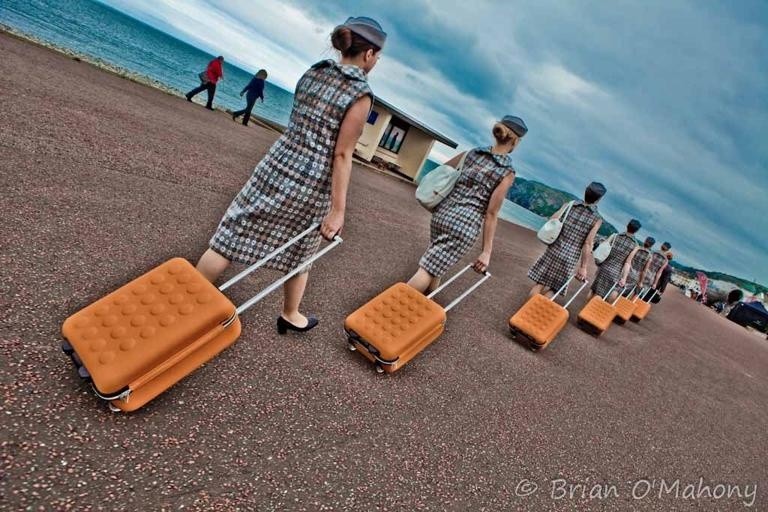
[277,316,318,334]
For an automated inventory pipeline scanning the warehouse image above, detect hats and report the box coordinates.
[502,115,528,137]
[589,182,606,195]
[344,16,386,48]
[631,220,641,229]
[664,242,671,248]
[647,237,655,244]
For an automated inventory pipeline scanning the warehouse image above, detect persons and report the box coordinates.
[607,237,656,303]
[583,218,642,303]
[185,55,225,111]
[678,284,727,314]
[232,69,268,126]
[526,181,608,300]
[196,16,387,334]
[630,241,672,301]
[651,252,673,295]
[407,115,527,294]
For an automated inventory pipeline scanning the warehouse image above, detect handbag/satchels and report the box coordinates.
[415,165,458,210]
[537,218,562,244]
[593,241,611,264]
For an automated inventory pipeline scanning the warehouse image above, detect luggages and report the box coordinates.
[509,272,658,352]
[62,224,344,413]
[344,263,491,373]
